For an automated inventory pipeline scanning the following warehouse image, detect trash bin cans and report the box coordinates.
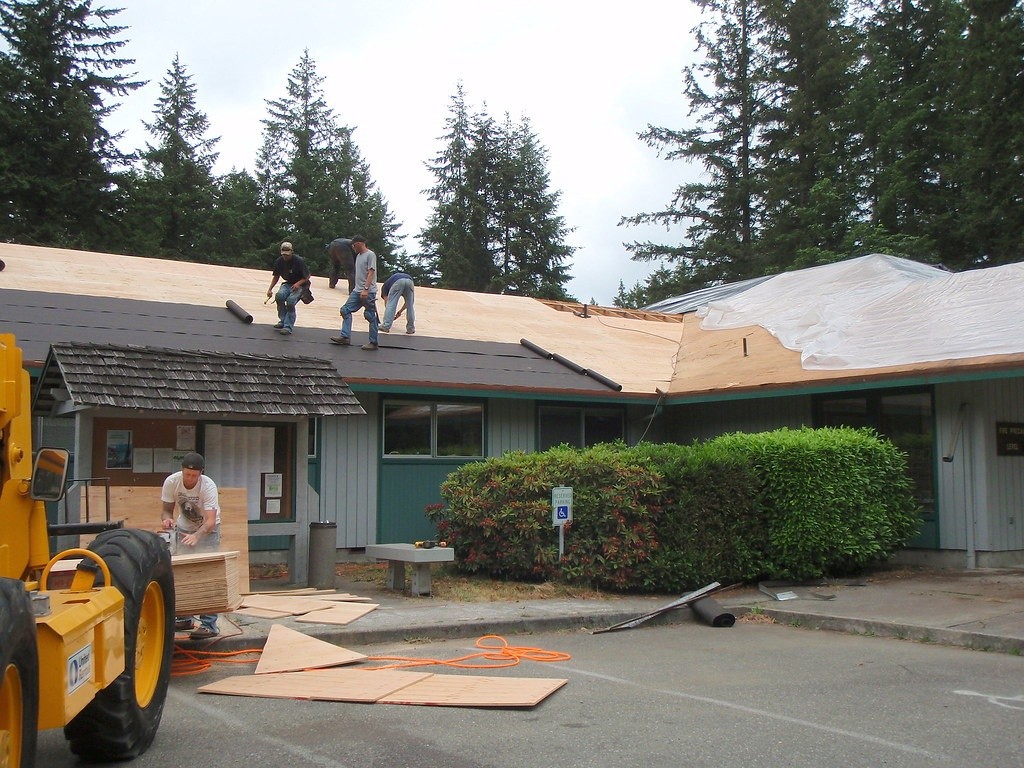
[308,521,338,590]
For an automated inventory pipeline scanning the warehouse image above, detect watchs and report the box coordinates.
[364,286,369,290]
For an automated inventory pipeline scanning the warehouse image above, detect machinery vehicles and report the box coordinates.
[0,330,179,768]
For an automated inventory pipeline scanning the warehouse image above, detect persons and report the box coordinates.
[330,235,379,350]
[378,273,415,334]
[267,242,311,335]
[160,451,221,639]
[327,238,359,295]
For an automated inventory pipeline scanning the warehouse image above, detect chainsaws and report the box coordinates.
[157,522,180,556]
[414,540,447,549]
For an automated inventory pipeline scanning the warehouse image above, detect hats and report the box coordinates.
[351,234,366,243]
[182,453,205,471]
[281,242,293,255]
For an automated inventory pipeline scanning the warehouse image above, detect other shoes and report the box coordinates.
[174,620,194,630]
[191,625,217,638]
[274,322,284,328]
[361,342,379,351]
[379,325,390,334]
[330,336,351,345]
[329,283,335,288]
[406,330,414,334]
[280,326,292,335]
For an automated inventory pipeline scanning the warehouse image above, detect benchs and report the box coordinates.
[364,543,454,598]
